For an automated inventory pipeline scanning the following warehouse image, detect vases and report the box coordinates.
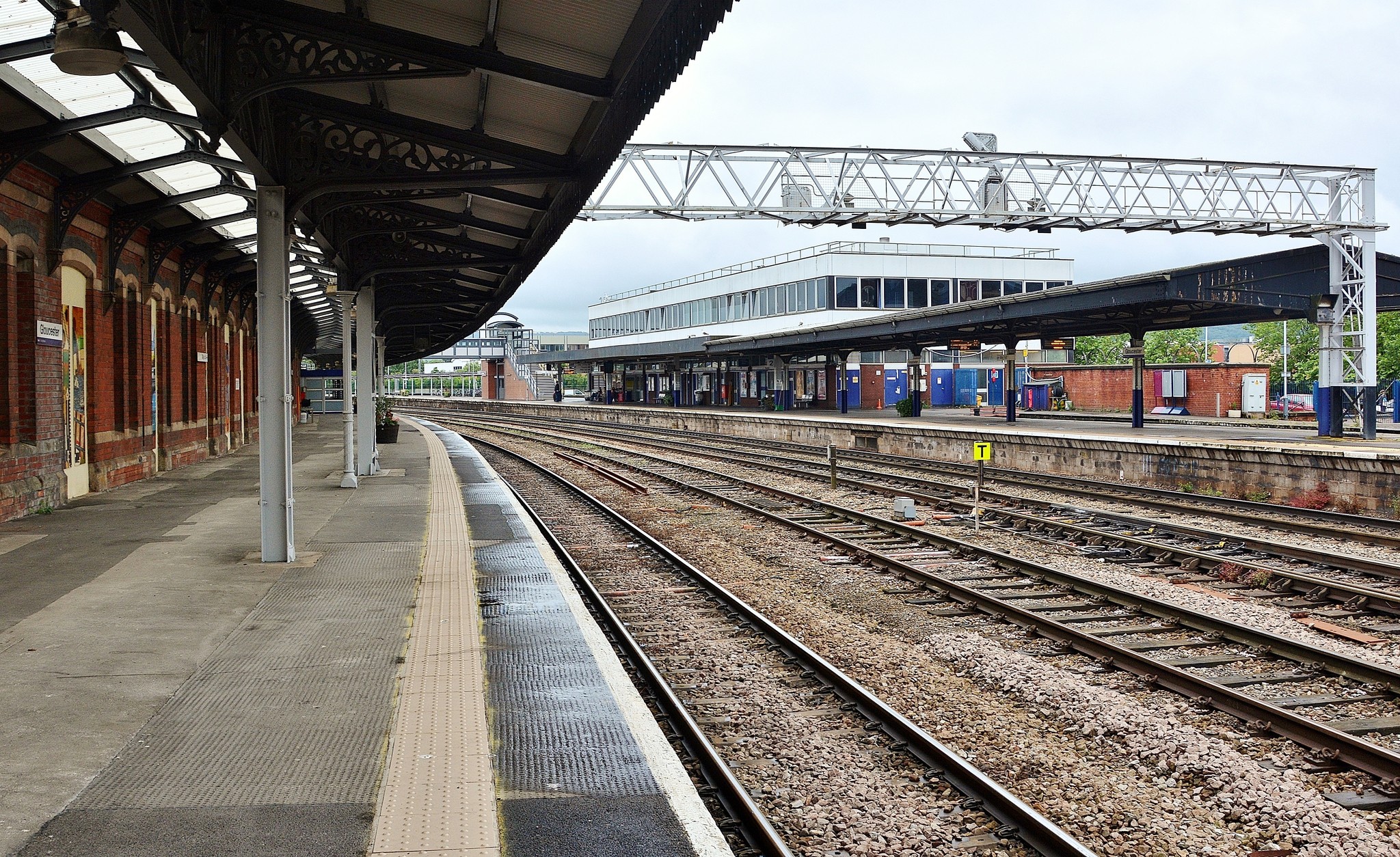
[376,424,400,443]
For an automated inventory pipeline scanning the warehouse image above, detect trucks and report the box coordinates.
[563,389,585,396]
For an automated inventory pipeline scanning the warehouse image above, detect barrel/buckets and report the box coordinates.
[300,412,307,423]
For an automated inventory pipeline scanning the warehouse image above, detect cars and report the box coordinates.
[1270,394,1313,411]
[1376,399,1394,414]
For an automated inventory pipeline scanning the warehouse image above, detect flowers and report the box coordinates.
[375,394,400,426]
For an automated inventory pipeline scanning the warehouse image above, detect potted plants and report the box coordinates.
[602,392,607,404]
[765,396,775,410]
[663,388,673,407]
[1228,401,1241,418]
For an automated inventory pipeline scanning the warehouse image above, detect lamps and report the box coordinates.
[50,26,128,76]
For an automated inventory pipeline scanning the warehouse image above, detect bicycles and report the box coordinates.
[432,387,482,397]
